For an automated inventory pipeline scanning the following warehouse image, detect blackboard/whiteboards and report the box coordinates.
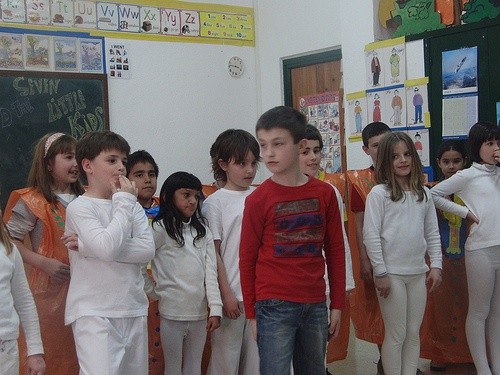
[0,69,111,218]
[0,24,266,198]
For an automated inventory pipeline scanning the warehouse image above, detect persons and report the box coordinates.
[3,130,80,375]
[197,128,263,375]
[427,140,476,372]
[347,121,424,375]
[60,131,157,375]
[239,106,346,375]
[430,120,500,375]
[362,132,443,375]
[140,171,223,375]
[290,124,355,375]
[0,210,46,375]
[126,150,164,375]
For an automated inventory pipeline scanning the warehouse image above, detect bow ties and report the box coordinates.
[144,205,160,220]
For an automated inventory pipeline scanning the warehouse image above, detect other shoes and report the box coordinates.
[416,368,424,375]
[373,359,385,375]
[326,368,333,375]
[430,360,447,371]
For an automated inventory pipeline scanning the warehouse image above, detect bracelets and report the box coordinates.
[380,273,387,276]
